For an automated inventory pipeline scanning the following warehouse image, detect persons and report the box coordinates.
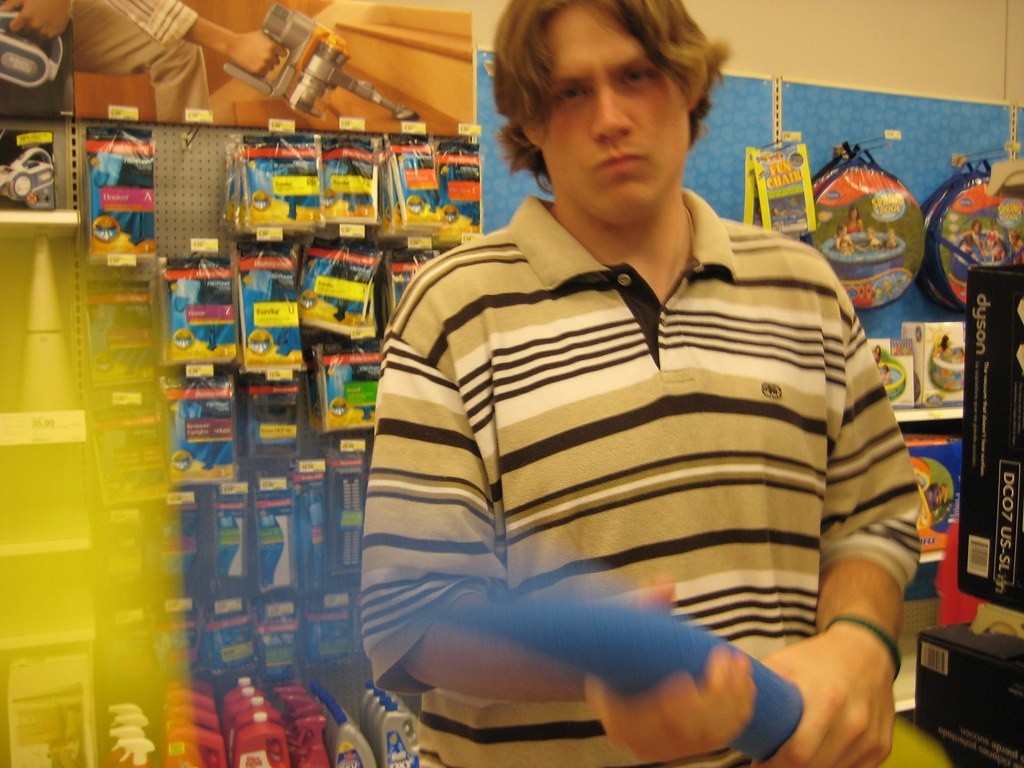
[835,204,896,255]
[874,345,881,363]
[359,0,922,768]
[71,1,288,123]
[932,335,952,357]
[2,0,71,41]
[881,365,890,384]
[960,218,1024,265]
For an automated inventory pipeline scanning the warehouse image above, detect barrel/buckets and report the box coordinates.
[162,675,419,768]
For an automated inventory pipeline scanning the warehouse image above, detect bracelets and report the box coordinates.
[824,613,902,683]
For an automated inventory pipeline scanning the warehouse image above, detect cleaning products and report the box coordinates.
[99,703,155,768]
[222,677,291,768]
[359,680,419,768]
[310,681,377,768]
[160,676,228,768]
[270,686,331,768]
